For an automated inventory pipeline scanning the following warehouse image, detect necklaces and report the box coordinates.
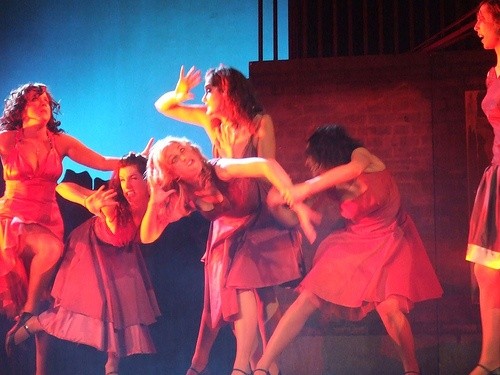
[34,133,44,155]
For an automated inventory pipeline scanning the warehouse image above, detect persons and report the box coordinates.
[465,0,500,375]
[140,65,443,375]
[5,153,161,374]
[0,84,153,375]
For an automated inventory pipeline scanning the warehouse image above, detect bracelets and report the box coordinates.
[84,198,87,206]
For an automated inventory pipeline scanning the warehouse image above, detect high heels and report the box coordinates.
[5,312,35,359]
